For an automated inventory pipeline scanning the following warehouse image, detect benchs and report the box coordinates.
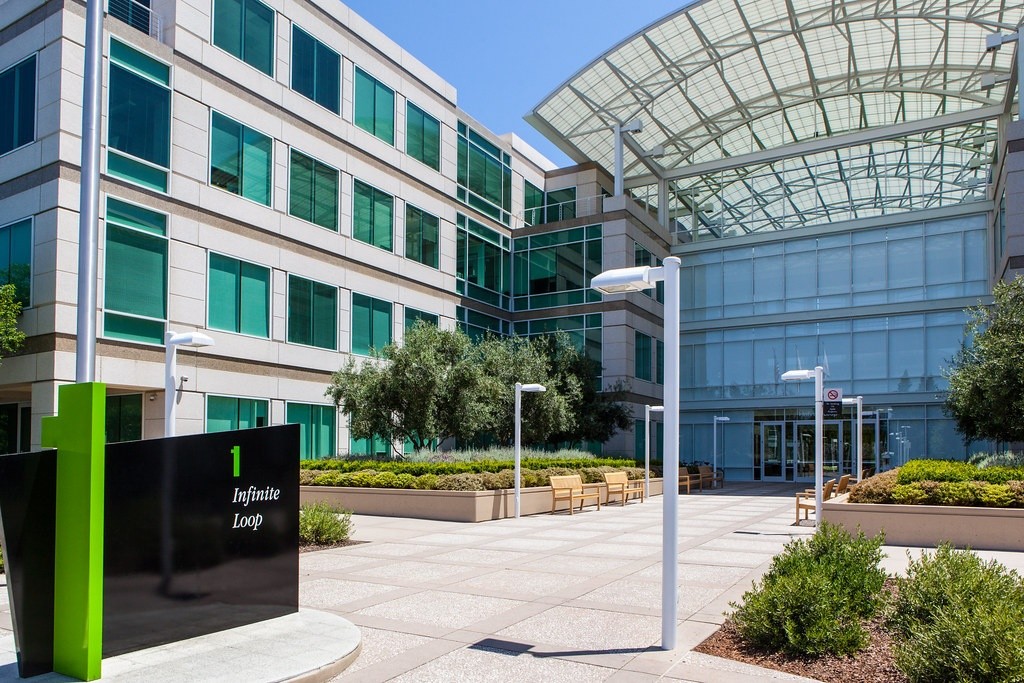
[795,480,835,525]
[604,471,644,506]
[698,466,724,491]
[847,467,876,486]
[678,467,703,494]
[550,475,600,515]
[815,475,849,497]
